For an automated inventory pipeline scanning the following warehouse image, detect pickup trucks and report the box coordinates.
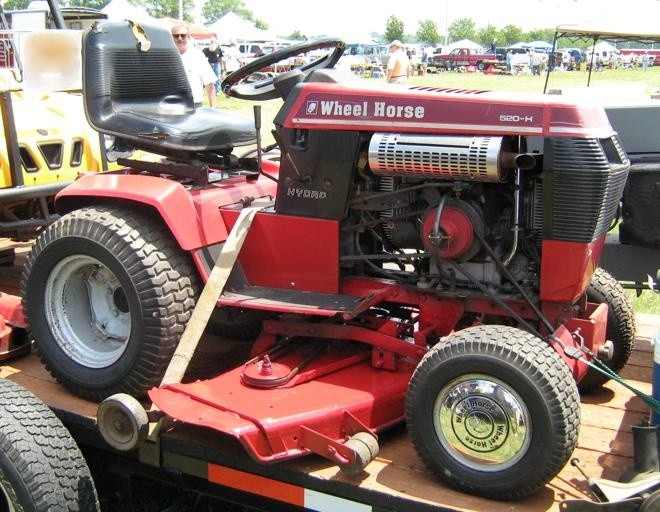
[426,45,500,71]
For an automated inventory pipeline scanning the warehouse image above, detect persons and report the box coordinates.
[171,24,219,111]
[226,37,245,74]
[351,37,652,78]
[383,41,414,87]
[202,39,225,99]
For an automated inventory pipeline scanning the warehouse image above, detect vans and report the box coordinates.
[233,37,388,73]
[483,46,582,69]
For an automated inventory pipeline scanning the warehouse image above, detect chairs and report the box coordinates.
[81,19,262,152]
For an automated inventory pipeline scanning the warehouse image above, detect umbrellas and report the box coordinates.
[160,14,198,32]
[102,0,157,26]
[203,11,276,47]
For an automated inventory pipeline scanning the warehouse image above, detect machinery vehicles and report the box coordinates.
[14,13,646,503]
[0,0,141,194]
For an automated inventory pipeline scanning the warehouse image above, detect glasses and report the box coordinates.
[172,34,187,39]
